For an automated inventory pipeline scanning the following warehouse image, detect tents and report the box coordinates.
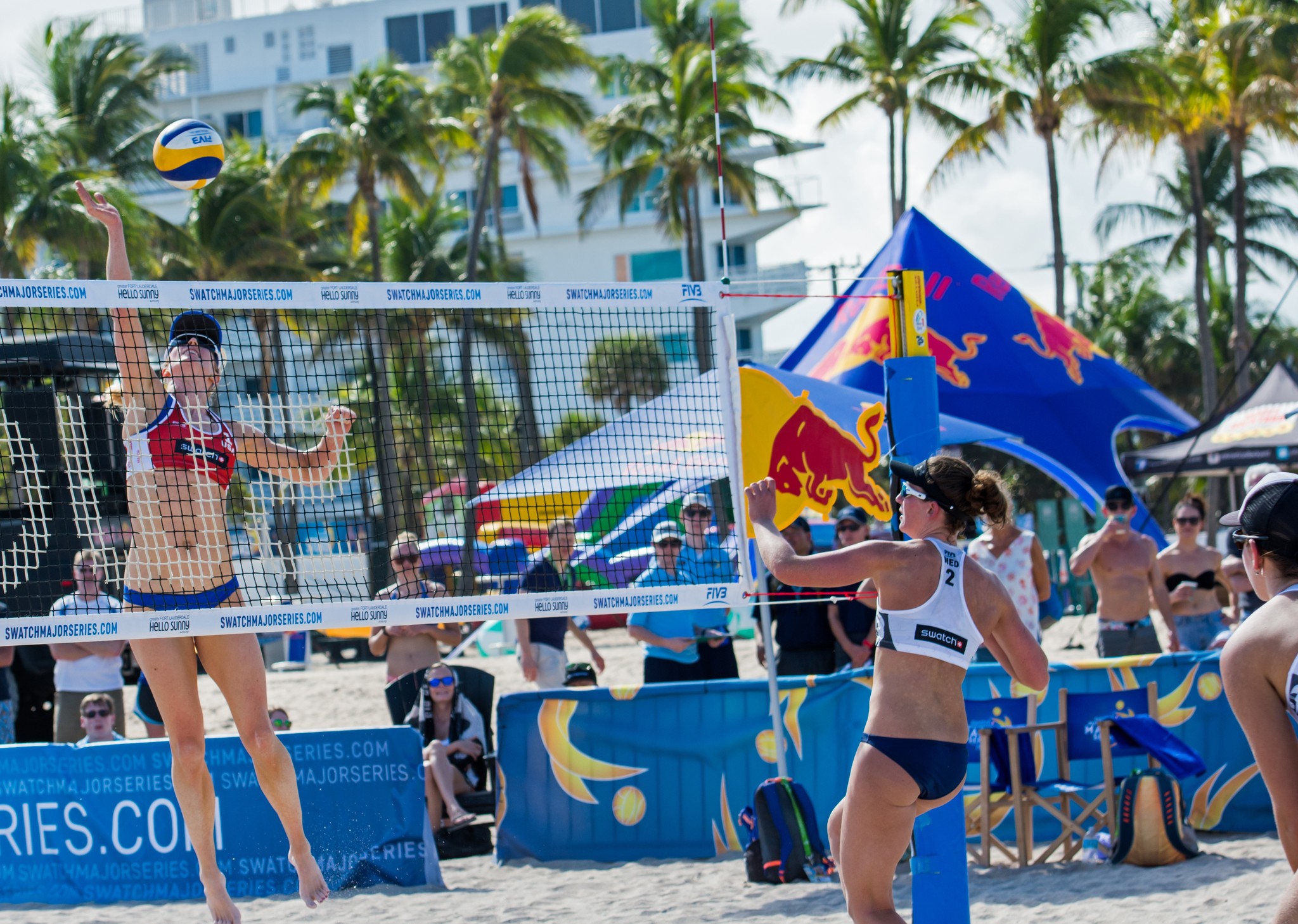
[1121,361,1298,510]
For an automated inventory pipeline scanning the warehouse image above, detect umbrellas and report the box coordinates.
[462,356,1023,778]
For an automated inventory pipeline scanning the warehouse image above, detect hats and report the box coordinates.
[1105,485,1132,503]
[1218,472,1298,564]
[681,491,711,510]
[562,665,597,685]
[170,311,222,353]
[834,506,867,525]
[791,517,810,532]
[651,520,681,543]
[890,459,976,531]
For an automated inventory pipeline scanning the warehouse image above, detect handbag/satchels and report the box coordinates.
[434,825,494,860]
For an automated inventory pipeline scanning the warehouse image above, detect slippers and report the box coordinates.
[447,811,477,832]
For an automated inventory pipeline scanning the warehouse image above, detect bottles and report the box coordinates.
[1082,826,1099,869]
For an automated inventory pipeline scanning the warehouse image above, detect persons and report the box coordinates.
[751,462,1280,676]
[513,518,606,688]
[562,662,601,688]
[404,664,488,832]
[368,532,462,726]
[133,671,168,746]
[268,706,292,731]
[628,490,740,684]
[78,693,129,743]
[744,456,1050,924]
[0,602,19,745]
[75,181,358,924]
[47,550,126,743]
[1218,472,1298,924]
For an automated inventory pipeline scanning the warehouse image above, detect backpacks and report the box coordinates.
[738,776,834,886]
[1109,769,1198,864]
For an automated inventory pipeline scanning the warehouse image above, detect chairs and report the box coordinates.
[385,663,494,861]
[964,682,1157,871]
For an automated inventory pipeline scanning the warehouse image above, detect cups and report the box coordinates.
[1182,581,1198,601]
[1114,513,1127,534]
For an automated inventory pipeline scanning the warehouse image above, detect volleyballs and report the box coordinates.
[151,118,224,190]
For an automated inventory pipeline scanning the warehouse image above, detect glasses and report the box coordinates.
[658,539,682,548]
[840,522,859,532]
[1107,501,1132,511]
[1232,528,1269,551]
[901,482,954,510]
[394,554,419,564]
[1176,517,1198,526]
[564,661,592,672]
[168,336,220,366]
[685,508,711,518]
[85,708,109,719]
[429,676,454,687]
[272,719,292,728]
[78,567,97,573]
